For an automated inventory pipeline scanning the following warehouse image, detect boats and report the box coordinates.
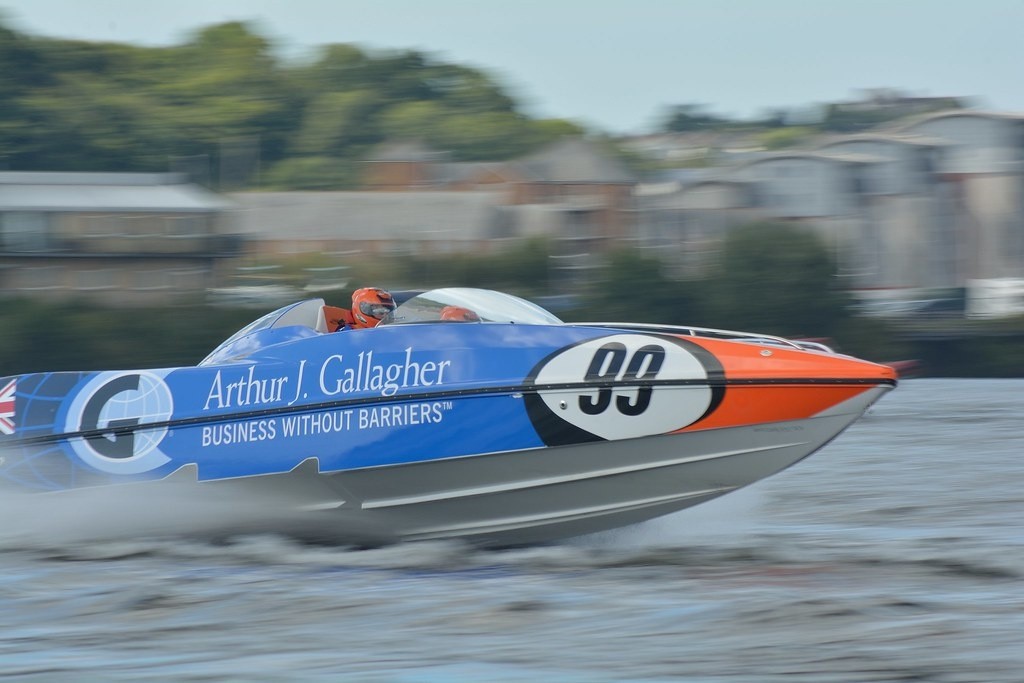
[1,286,899,554]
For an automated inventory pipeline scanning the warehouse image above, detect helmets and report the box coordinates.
[441,306,482,322]
[351,287,397,328]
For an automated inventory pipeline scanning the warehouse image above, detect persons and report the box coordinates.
[336,287,397,331]
[439,305,482,321]
